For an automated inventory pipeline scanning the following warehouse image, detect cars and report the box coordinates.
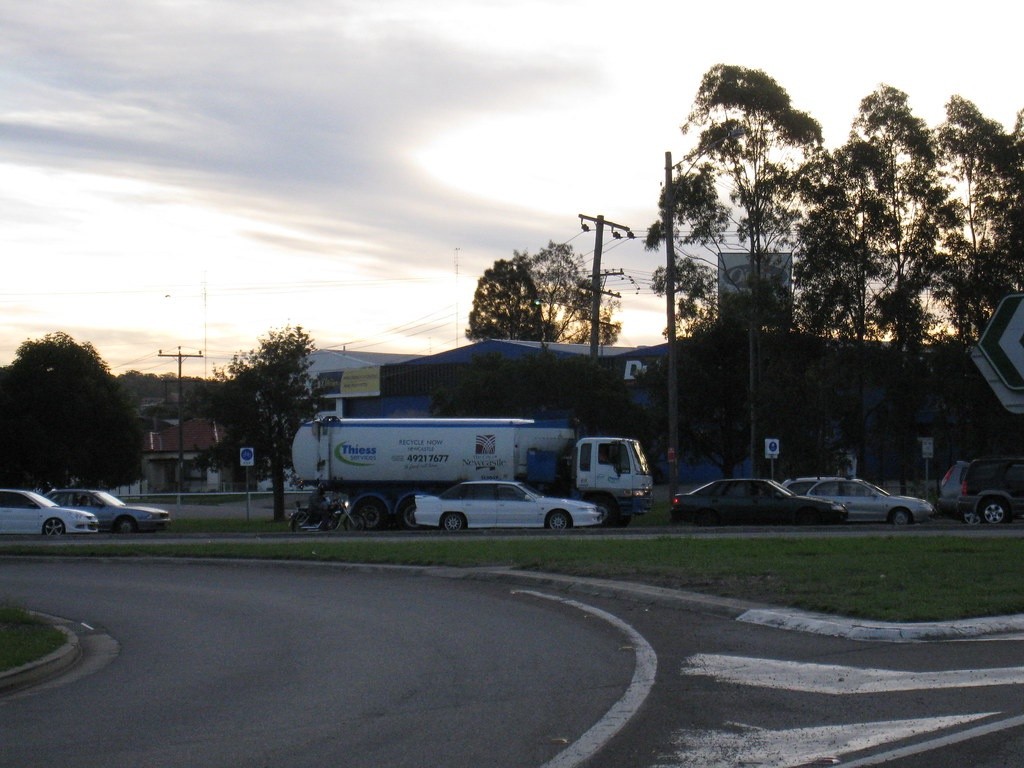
[0,488,99,537]
[670,478,848,526]
[413,478,604,534]
[780,475,937,525]
[42,489,174,535]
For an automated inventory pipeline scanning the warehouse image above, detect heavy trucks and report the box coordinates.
[291,412,653,530]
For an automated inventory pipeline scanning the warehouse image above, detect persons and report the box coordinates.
[308,486,333,521]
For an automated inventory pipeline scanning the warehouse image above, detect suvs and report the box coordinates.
[936,461,968,523]
[958,455,1024,526]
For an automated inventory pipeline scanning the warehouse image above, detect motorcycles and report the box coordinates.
[287,491,367,534]
